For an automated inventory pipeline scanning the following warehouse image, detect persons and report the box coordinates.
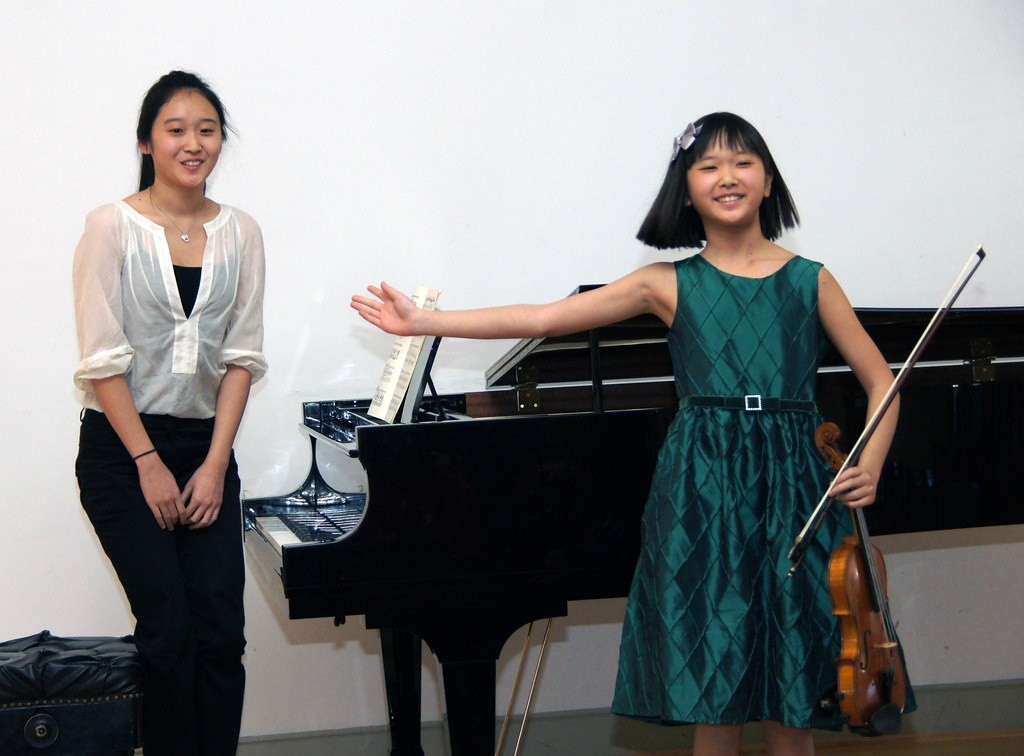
[72,70,269,756]
[352,112,918,756]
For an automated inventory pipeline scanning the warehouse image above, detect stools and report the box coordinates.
[0,630,148,756]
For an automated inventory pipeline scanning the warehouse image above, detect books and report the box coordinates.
[366,289,440,424]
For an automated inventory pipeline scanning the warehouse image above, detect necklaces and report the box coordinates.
[148,186,204,242]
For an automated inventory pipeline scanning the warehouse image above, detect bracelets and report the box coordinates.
[133,448,155,461]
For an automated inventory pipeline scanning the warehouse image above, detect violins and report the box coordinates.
[815,421,907,737]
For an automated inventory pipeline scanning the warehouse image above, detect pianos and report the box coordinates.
[239,285,1024,756]
